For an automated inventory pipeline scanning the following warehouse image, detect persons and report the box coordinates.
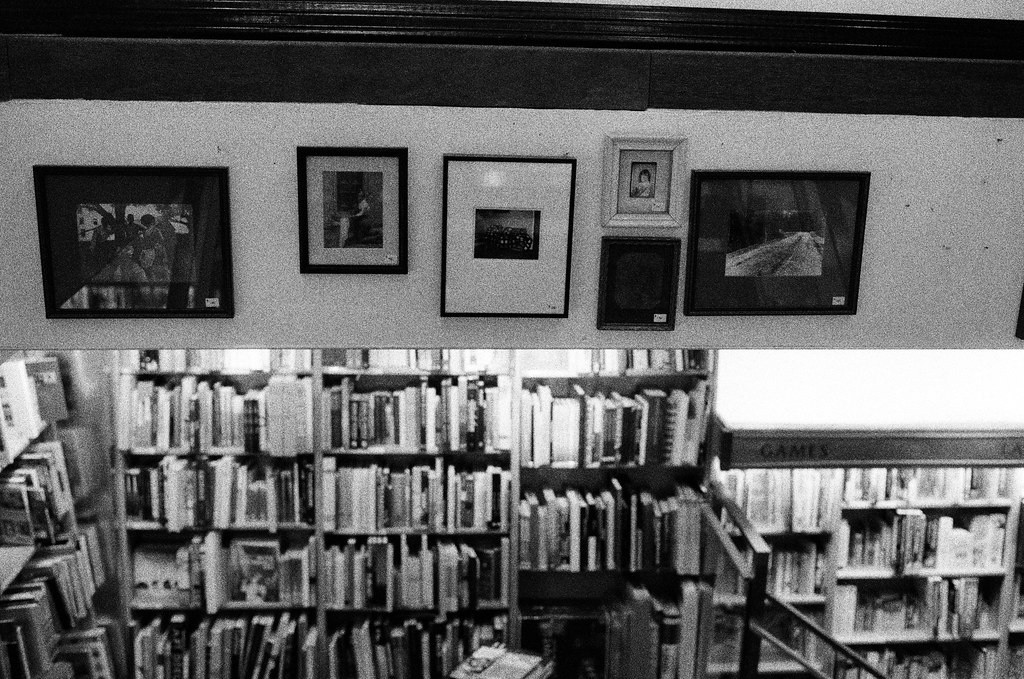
[350,191,383,246]
[89,212,170,307]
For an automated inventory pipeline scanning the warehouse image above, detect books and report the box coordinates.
[715,464,1024,679]
[0,350,716,679]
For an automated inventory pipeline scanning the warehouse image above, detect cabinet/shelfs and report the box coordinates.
[0,349,1024,679]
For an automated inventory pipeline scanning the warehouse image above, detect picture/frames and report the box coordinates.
[602,132,687,230]
[440,154,578,319]
[597,235,682,332]
[683,170,873,317]
[296,146,411,274]
[34,164,237,318]
